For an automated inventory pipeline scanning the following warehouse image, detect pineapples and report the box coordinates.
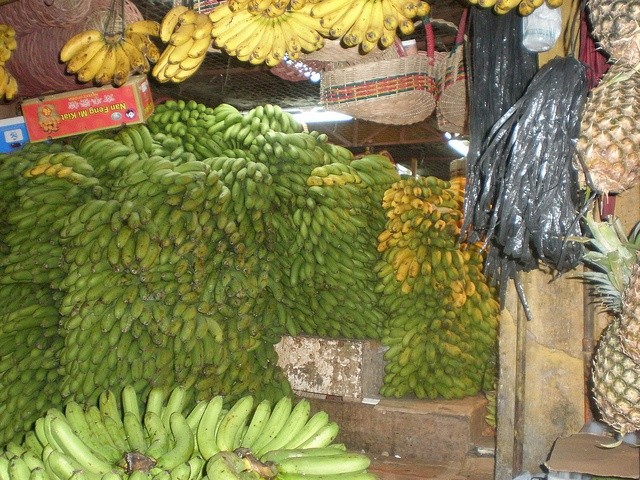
[585,1,640,68]
[566,201,640,435]
[573,64,640,195]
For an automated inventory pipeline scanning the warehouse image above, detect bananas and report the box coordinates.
[313,0,427,55]
[210,0,330,66]
[1,21,21,105]
[466,1,562,16]
[153,6,212,83]
[60,19,162,87]
[1,384,379,479]
[1,100,498,451]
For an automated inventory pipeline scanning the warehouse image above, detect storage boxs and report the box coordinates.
[22,75,154,144]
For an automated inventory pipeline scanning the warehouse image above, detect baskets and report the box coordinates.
[270,55,323,83]
[439,9,472,128]
[298,35,399,63]
[318,15,437,127]
